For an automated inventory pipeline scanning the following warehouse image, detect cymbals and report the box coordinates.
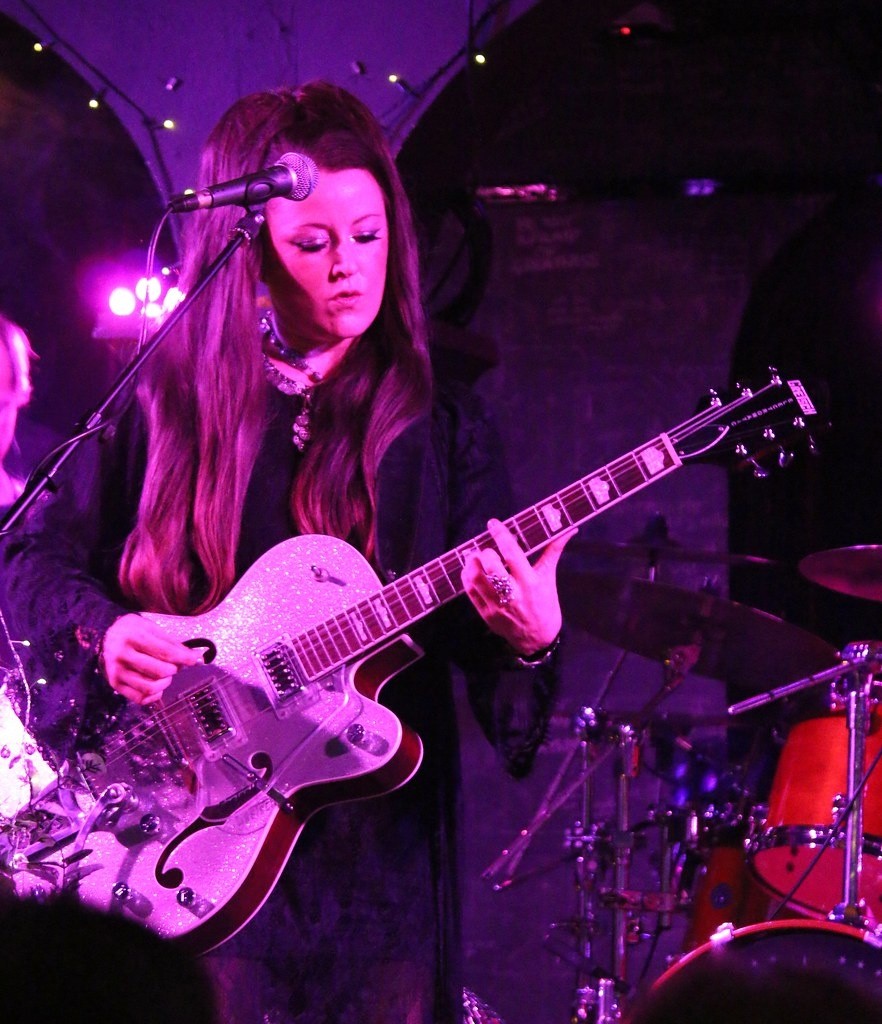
[800,544,882,601]
[572,537,774,565]
[552,580,842,701]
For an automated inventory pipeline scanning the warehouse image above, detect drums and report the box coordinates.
[687,847,781,952]
[746,708,882,941]
[627,917,882,1023]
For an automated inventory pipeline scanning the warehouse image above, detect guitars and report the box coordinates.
[0,362,830,959]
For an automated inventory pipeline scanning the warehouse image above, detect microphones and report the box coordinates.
[172,153,315,207]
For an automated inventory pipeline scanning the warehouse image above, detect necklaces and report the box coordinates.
[259,308,322,452]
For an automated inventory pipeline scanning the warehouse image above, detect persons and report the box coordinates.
[1,79,579,1022]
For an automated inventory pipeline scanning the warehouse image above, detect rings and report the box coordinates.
[482,571,515,607]
